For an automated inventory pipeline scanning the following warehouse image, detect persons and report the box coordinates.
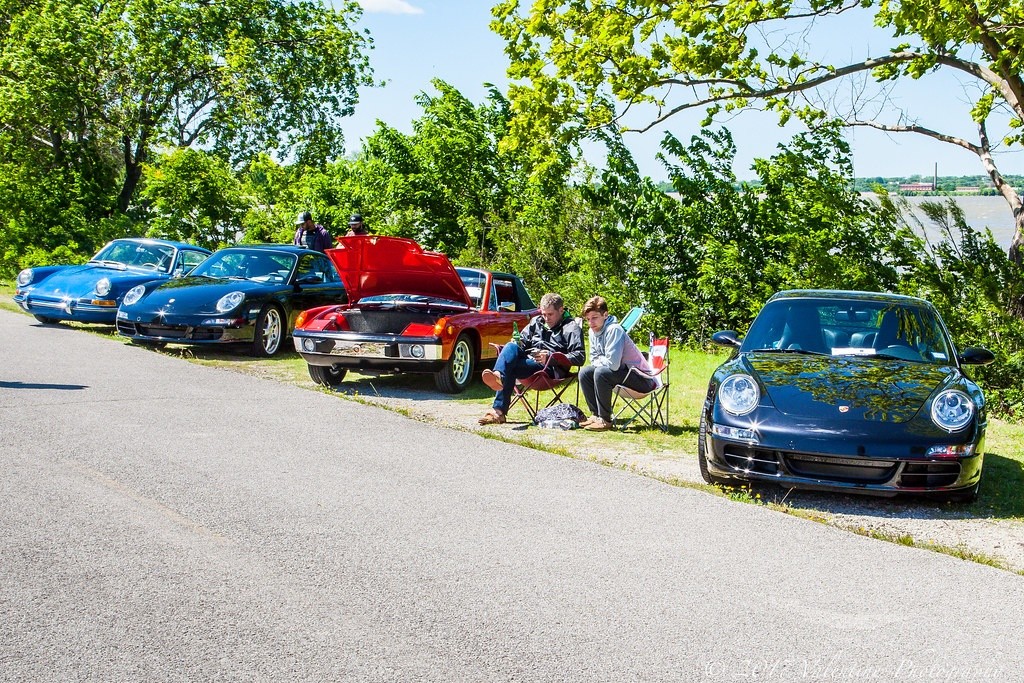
[293,214,368,253]
[476,293,586,424]
[576,295,660,432]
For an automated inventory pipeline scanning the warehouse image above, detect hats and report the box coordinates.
[347,214,362,224]
[295,212,311,225]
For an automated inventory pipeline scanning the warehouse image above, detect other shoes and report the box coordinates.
[479,409,506,424]
[585,417,612,431]
[579,415,599,427]
[482,369,503,391]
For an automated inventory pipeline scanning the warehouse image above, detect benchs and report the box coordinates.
[820,324,883,348]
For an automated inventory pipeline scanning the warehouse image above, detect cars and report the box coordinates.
[114,242,346,358]
[12,236,245,327]
[696,287,996,505]
[291,234,568,394]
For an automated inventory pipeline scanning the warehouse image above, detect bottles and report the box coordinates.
[512,322,521,346]
[649,320,659,352]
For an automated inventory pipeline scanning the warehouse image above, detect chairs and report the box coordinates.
[776,304,929,362]
[620,306,645,334]
[489,317,582,425]
[610,331,670,433]
[243,259,272,279]
